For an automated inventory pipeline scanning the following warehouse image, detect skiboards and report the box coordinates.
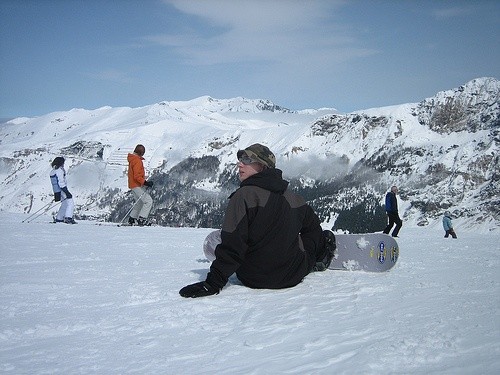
[95,223,158,228]
[34,221,79,225]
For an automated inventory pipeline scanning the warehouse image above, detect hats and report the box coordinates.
[134,144,146,156]
[236,143,276,169]
[444,211,451,216]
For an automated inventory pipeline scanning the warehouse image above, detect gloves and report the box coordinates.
[62,187,72,199]
[144,180,154,186]
[179,281,214,299]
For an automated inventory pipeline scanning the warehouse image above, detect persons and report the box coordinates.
[383,186,402,238]
[442,211,457,239]
[127,144,153,226]
[179,143,335,297]
[49,157,77,224]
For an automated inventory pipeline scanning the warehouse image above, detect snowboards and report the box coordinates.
[202,229,400,274]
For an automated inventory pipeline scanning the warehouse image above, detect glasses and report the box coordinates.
[239,156,255,165]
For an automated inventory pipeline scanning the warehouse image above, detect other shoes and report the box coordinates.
[138,218,151,226]
[64,217,78,224]
[128,217,138,225]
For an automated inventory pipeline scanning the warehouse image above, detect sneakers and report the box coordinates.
[314,230,337,271]
